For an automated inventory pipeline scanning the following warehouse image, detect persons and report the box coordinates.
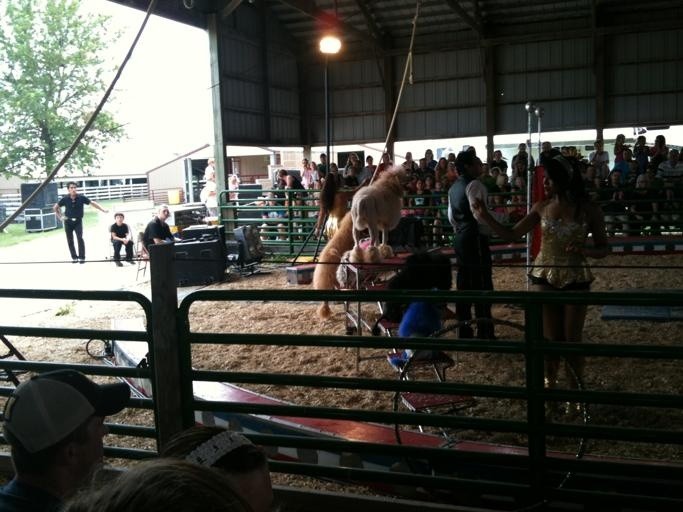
[472,152,610,417]
[1,368,132,511]
[447,145,496,340]
[65,460,256,512]
[257,147,507,243]
[143,204,173,253]
[200,157,240,225]
[110,212,136,267]
[160,424,274,510]
[53,181,110,264]
[313,170,369,305]
[511,135,682,247]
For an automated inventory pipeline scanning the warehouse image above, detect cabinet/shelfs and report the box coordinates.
[343,257,417,370]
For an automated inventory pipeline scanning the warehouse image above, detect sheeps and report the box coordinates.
[310,209,354,319]
[349,164,415,267]
[371,250,453,336]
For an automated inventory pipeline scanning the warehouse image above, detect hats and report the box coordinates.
[4,370,131,456]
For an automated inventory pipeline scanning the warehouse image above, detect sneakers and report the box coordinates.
[72,257,84,264]
[114,258,135,267]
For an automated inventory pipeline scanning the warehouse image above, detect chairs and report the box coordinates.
[137,233,154,281]
